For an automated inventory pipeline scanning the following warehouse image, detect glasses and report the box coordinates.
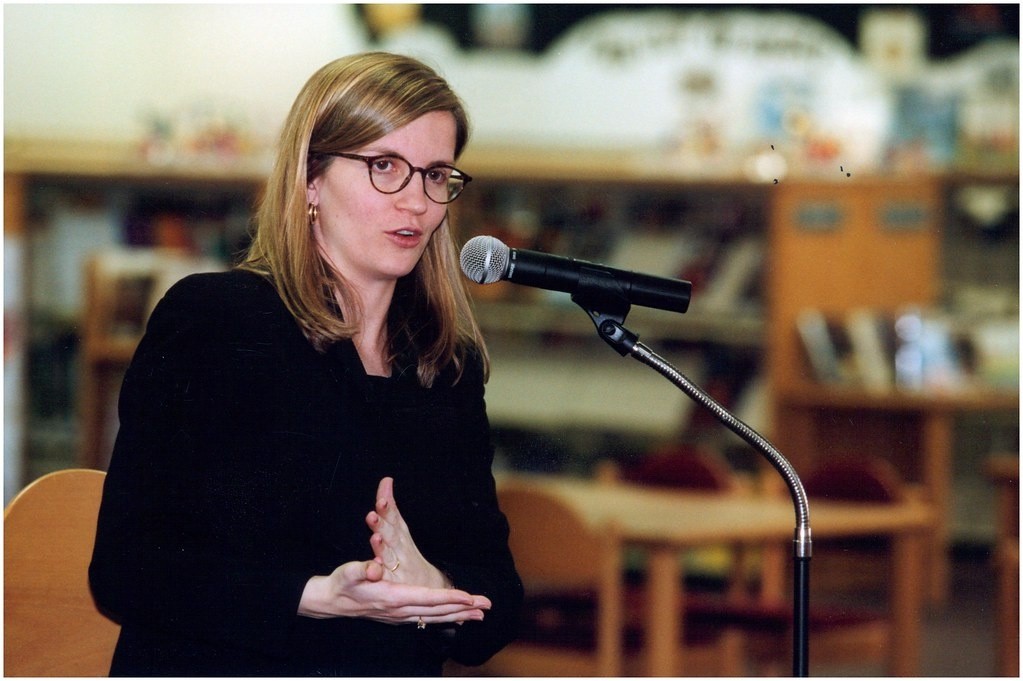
[311,151,472,205]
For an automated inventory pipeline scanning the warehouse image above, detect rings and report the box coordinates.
[418,616,426,629]
[391,561,400,571]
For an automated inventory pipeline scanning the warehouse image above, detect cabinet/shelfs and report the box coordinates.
[6,136,782,490]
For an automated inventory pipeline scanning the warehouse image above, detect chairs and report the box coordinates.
[449,487,631,677]
[4,467,125,677]
[773,173,1019,678]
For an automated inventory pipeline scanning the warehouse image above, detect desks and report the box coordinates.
[504,469,939,677]
[779,379,1019,678]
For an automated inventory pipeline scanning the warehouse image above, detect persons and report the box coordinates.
[88,52,523,678]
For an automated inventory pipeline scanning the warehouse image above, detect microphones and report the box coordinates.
[460,233,694,315]
